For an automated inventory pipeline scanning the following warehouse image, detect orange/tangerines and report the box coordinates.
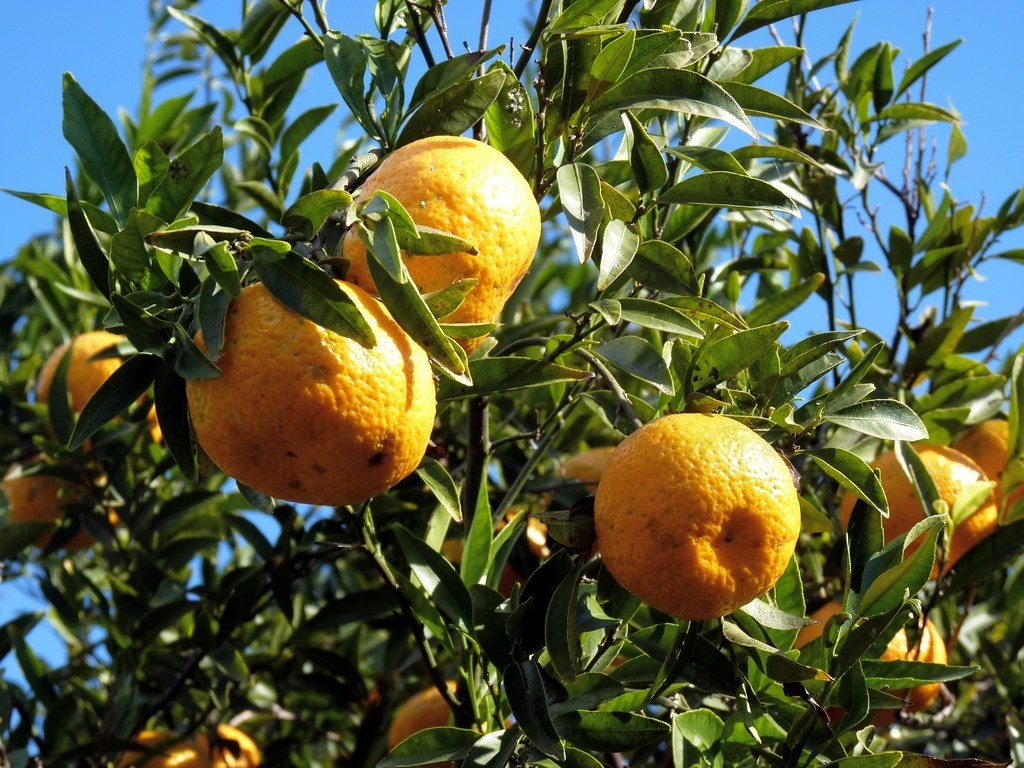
[0,136,1024,768]
